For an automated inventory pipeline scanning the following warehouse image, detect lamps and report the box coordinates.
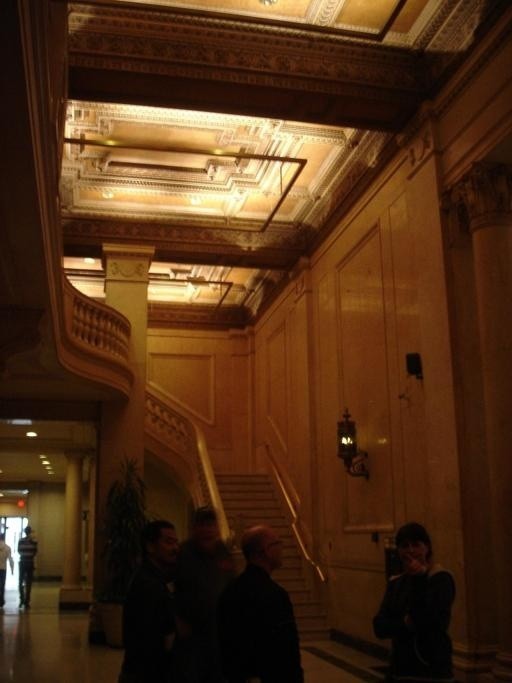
[337,408,368,477]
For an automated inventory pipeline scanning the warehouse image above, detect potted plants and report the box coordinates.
[97,450,151,647]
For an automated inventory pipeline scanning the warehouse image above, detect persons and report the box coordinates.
[117,517,182,682]
[0,532,15,608]
[17,524,38,610]
[369,520,459,681]
[213,522,308,682]
[171,503,235,682]
